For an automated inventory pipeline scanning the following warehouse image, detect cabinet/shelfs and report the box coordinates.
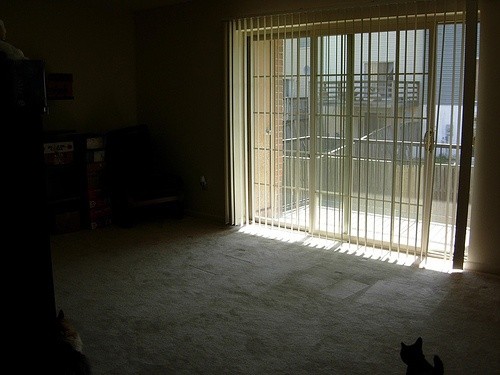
[39,131,116,232]
